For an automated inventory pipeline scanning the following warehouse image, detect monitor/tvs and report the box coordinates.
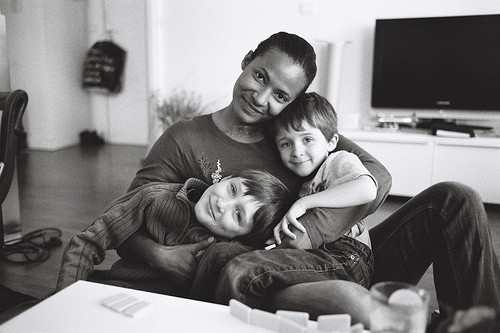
[371,14,500,111]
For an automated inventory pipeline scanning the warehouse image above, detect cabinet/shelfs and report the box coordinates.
[339,127,500,204]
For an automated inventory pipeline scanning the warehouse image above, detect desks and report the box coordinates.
[0,280,370,333]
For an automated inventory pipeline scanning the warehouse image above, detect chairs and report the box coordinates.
[0,89,29,251]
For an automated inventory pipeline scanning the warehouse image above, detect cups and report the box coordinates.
[368,281,430,333]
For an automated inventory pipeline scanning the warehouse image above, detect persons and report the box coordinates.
[189,92,378,310]
[88,32,500,333]
[56,168,291,298]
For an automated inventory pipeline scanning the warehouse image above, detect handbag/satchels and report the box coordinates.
[81,41,127,94]
